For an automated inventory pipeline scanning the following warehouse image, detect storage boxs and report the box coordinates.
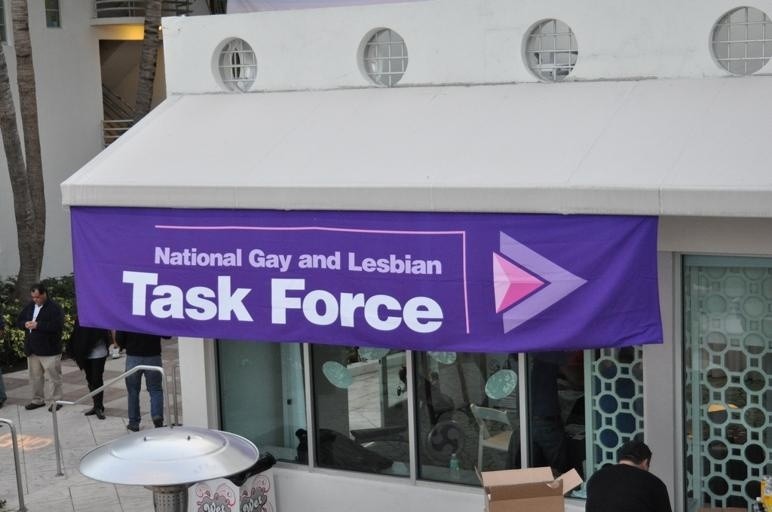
[475,463,585,512]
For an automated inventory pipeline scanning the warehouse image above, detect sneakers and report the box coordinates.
[154,419,163,427]
[127,425,139,432]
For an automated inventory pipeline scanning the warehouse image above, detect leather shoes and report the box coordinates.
[25,403,45,410]
[49,403,62,412]
[84,406,106,419]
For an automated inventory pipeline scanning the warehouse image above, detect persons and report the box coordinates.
[506,353,568,484]
[398,367,457,450]
[584,438,672,511]
[19,282,64,413]
[113,327,168,431]
[67,317,115,419]
[1,312,9,406]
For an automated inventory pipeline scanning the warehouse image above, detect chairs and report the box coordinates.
[460,401,515,470]
